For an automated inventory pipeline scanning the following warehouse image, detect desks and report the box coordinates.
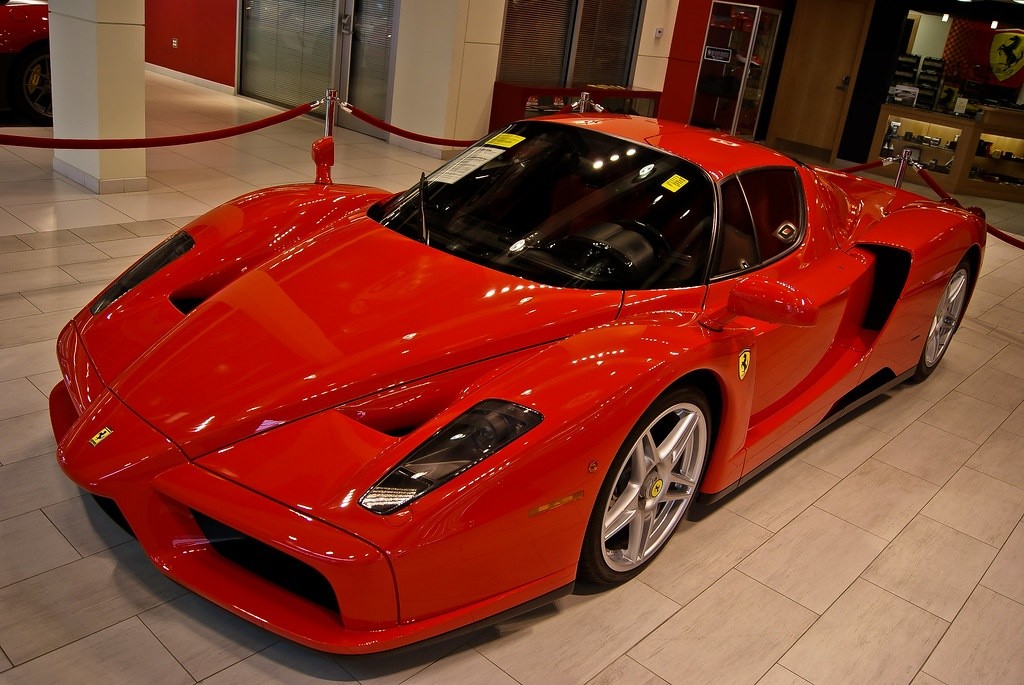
[488,81,663,133]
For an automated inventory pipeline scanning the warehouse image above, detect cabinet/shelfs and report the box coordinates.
[864,103,1024,203]
[887,54,946,111]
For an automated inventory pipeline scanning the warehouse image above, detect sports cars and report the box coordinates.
[0,0,53,128]
[47,124,984,659]
